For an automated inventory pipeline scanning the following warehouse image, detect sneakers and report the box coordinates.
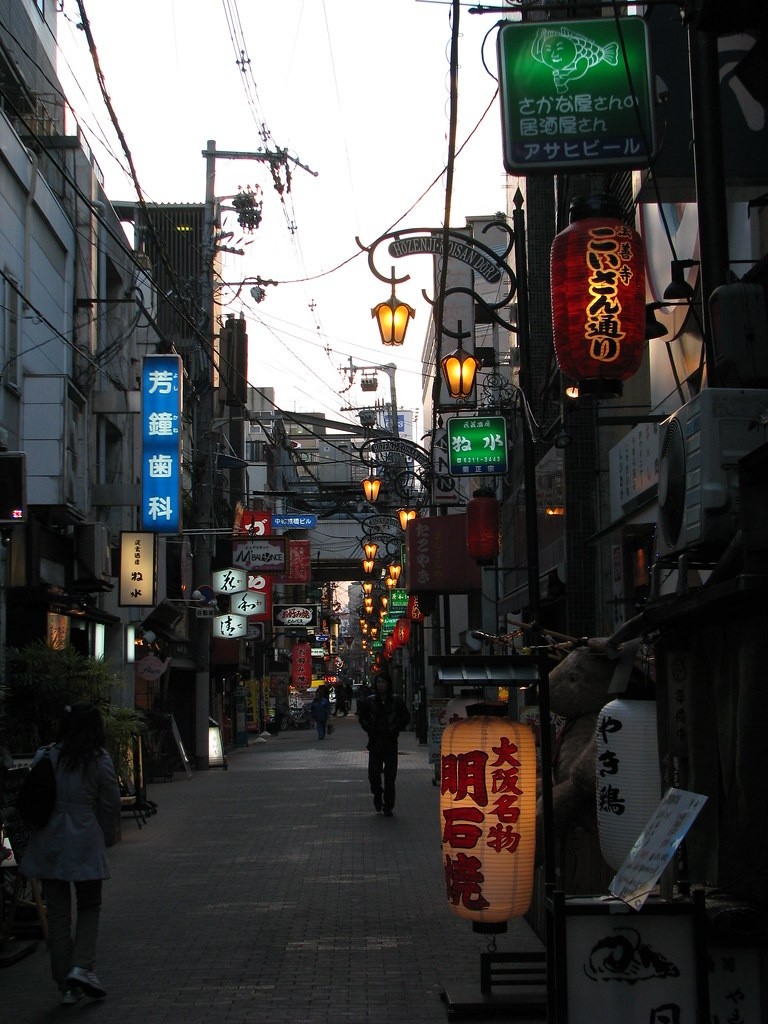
[65,967,107,998]
[61,990,80,1005]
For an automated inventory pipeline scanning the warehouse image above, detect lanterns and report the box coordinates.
[294,636,313,693]
[439,689,536,933]
[550,192,648,401]
[466,488,502,567]
[593,684,665,872]
[371,593,439,676]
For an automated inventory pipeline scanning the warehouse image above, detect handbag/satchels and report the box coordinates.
[15,755,57,829]
[327,721,335,734]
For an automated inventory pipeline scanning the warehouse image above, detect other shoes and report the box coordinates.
[382,807,393,817]
[318,736,324,740]
[332,712,337,716]
[373,789,383,812]
[342,711,349,716]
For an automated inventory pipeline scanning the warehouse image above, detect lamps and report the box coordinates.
[186,599,219,612]
[643,301,710,341]
[552,423,572,448]
[664,259,768,299]
[165,591,206,602]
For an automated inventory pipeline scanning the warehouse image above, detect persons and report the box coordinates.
[312,678,353,738]
[18,701,123,1007]
[360,673,411,816]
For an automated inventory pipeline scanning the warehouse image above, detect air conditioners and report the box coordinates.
[656,387,768,554]
[74,522,112,583]
[171,601,193,641]
